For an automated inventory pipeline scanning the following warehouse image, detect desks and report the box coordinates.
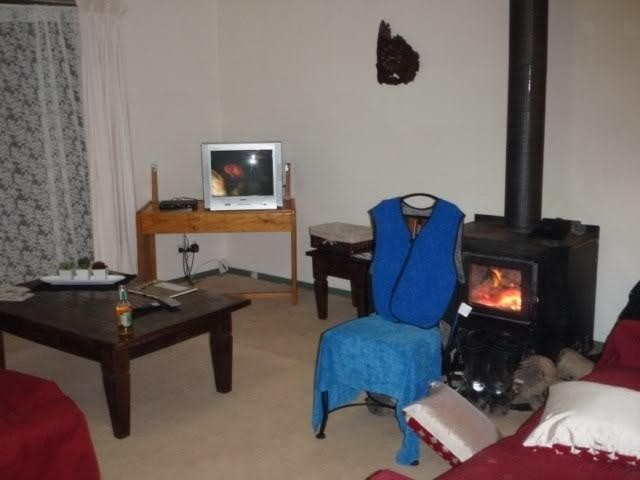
[137,198,297,305]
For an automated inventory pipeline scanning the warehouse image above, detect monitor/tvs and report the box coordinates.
[201,142,283,211]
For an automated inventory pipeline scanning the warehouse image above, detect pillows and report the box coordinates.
[524,376,639,471]
[404,382,505,467]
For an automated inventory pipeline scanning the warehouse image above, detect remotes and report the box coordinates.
[158,295,182,309]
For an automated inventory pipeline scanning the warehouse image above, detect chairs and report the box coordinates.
[316,193,462,465]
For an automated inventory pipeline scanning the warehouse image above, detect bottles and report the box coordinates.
[113,283,135,337]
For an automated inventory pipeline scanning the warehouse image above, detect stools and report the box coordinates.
[307,251,374,319]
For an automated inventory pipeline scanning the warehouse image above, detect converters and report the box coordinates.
[191,245,198,252]
